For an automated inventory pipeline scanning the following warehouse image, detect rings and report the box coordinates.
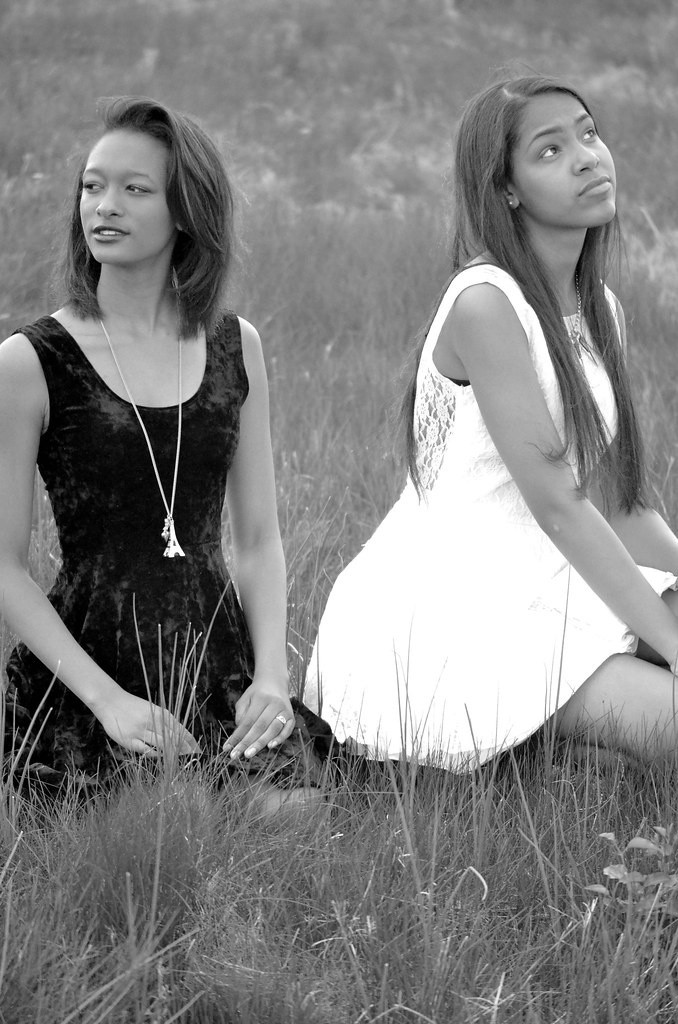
[276,715,287,724]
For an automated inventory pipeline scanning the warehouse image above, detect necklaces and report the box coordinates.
[97,313,188,559]
[569,272,599,372]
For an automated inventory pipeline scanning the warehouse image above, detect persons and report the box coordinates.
[0,98,343,814]
[300,77,678,777]
